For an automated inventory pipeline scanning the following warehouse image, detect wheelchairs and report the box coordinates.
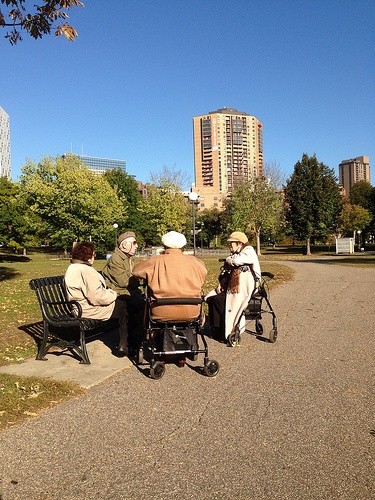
[143,278,220,380]
[201,259,278,347]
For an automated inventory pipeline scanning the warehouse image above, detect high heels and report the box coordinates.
[117,346,139,357]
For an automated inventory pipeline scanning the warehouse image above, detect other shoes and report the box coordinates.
[175,355,186,367]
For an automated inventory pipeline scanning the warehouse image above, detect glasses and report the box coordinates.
[126,241,137,246]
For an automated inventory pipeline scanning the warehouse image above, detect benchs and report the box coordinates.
[28,270,118,364]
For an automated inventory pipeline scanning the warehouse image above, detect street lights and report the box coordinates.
[188,191,199,257]
[113,222,119,251]
[357,230,362,251]
[200,222,204,248]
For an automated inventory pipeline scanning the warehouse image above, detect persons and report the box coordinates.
[101,230,141,294]
[64,241,138,356]
[204,231,261,341]
[132,231,208,361]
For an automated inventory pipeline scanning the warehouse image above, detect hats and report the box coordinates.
[118,231,136,244]
[227,231,248,245]
[161,231,187,249]
